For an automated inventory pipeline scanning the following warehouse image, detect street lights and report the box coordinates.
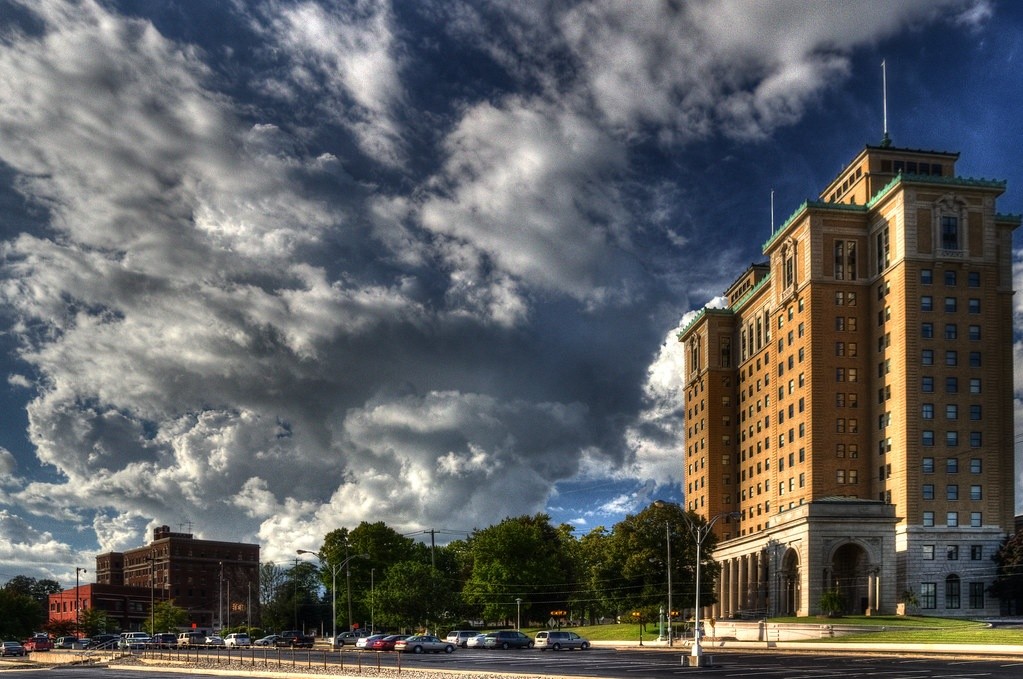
[515,598,524,631]
[222,577,230,630]
[144,556,155,636]
[653,499,743,656]
[76,567,87,642]
[295,549,366,652]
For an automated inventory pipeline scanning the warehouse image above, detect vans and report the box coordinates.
[447,630,481,648]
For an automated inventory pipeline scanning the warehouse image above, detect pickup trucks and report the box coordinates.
[23,636,55,654]
[82,635,116,649]
[273,630,314,649]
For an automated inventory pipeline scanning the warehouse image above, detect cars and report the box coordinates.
[356,633,388,649]
[0,642,24,657]
[372,634,414,649]
[466,633,487,648]
[533,631,590,651]
[53,636,76,648]
[223,632,251,649]
[253,635,280,646]
[483,630,536,649]
[145,633,178,649]
[176,631,205,649]
[393,635,457,654]
[205,637,221,645]
[326,631,367,647]
[118,632,153,650]
